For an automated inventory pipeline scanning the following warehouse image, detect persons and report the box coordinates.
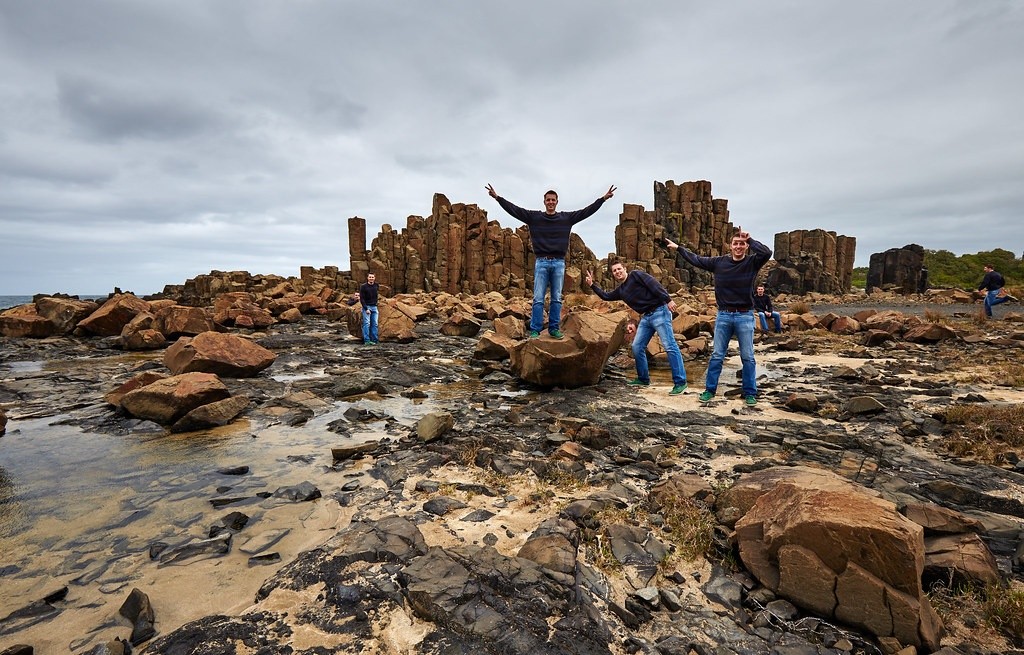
[664,225,773,406]
[484,183,618,340]
[753,285,781,336]
[978,264,1018,320]
[359,272,379,346]
[586,257,688,396]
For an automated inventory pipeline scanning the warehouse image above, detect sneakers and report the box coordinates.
[745,395,757,406]
[549,329,564,339]
[627,378,651,386]
[530,330,538,339]
[698,391,714,403]
[669,382,688,396]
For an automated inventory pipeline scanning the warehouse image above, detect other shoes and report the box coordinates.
[365,342,375,345]
[376,342,382,345]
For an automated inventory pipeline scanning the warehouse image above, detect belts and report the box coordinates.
[542,256,555,258]
[724,309,748,312]
